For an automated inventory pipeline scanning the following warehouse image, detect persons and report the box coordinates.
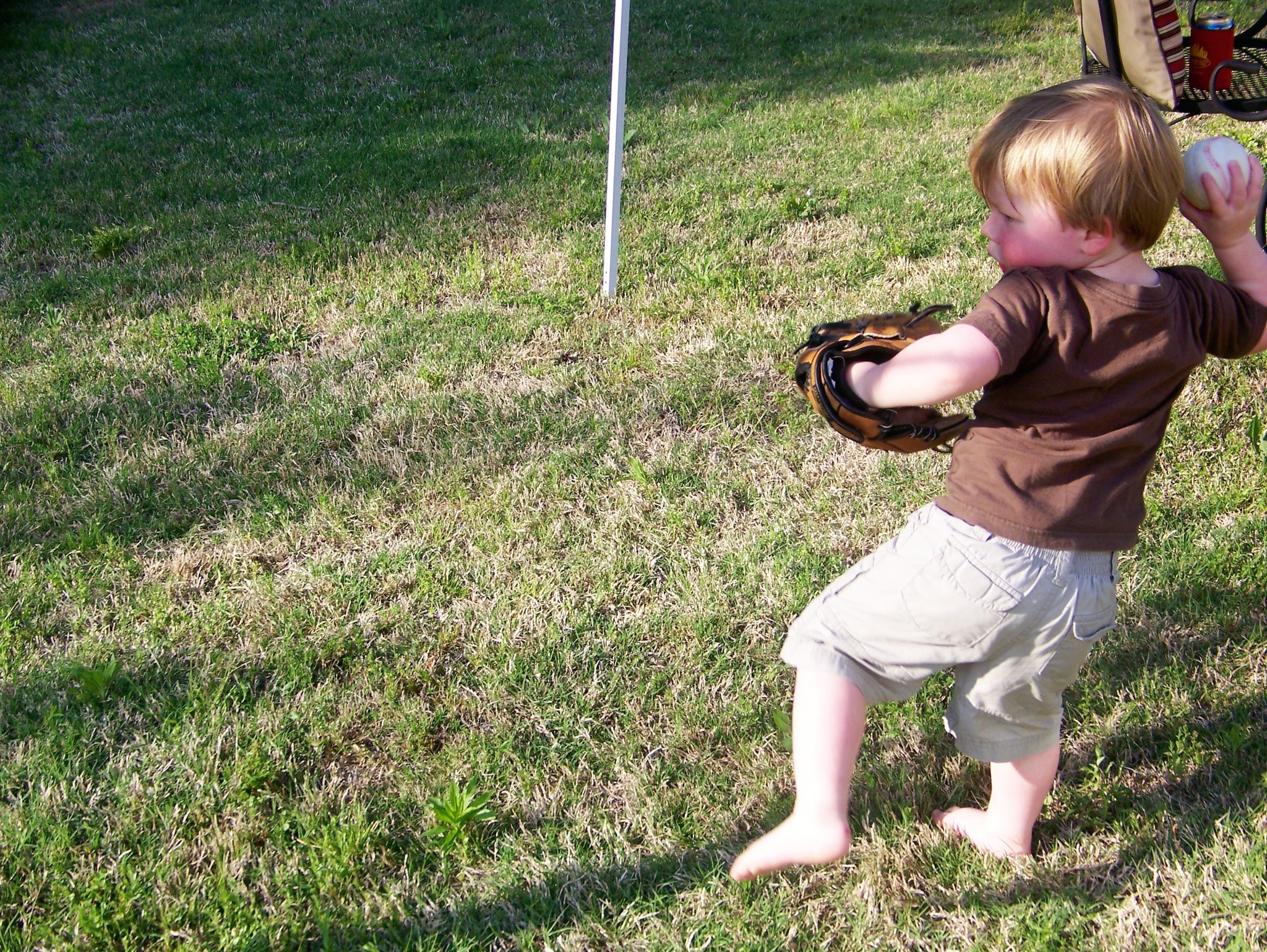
[729,76,1267,885]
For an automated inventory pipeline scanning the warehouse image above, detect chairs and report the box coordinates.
[1081,0,1267,254]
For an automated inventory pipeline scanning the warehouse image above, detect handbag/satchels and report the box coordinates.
[1074,0,1186,110]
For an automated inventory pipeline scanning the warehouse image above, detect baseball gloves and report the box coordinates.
[795,311,971,454]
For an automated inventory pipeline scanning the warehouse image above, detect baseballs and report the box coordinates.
[1181,135,1251,211]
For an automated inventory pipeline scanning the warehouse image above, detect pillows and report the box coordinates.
[1073,0,1186,110]
